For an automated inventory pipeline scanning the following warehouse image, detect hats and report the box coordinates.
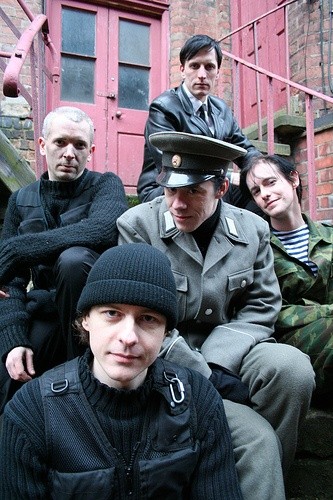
[76,243,181,330]
[147,130,247,189]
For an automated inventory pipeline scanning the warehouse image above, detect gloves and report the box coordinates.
[210,369,252,404]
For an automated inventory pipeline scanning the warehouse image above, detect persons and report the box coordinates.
[118,132,309,500]
[0,243,246,500]
[136,35,272,222]
[241,154,333,409]
[0,106,128,416]
